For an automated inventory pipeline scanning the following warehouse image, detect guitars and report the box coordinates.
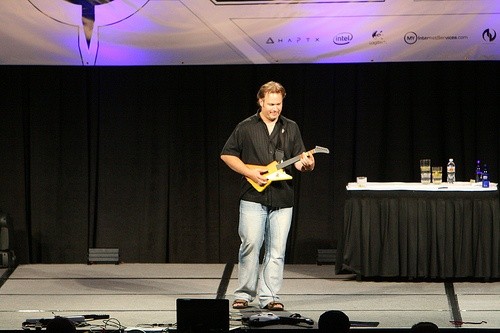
[244,145,330,193]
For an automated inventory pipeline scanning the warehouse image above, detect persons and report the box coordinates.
[220,81,315,312]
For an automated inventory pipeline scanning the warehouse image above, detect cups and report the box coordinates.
[356,177,367,188]
[420,159,431,185]
[432,166,443,185]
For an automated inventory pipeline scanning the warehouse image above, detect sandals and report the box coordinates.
[232,299,248,309]
[267,301,284,310]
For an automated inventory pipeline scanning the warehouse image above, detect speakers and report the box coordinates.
[176,298,230,333]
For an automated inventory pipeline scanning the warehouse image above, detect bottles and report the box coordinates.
[475,160,483,183]
[482,164,490,188]
[447,158,456,185]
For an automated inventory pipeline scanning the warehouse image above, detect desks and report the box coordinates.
[335,182,500,282]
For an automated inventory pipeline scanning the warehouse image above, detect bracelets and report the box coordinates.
[301,166,306,174]
